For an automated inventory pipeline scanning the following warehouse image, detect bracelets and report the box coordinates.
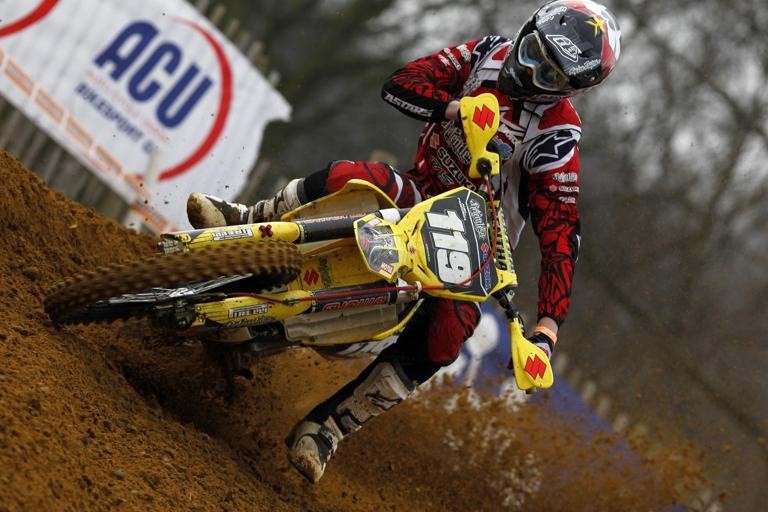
[533,326,558,346]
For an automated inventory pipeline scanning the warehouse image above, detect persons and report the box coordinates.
[186,0,622,486]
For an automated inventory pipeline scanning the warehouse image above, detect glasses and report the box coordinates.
[517,29,569,91]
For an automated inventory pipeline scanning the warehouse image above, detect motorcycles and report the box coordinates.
[47,88,558,394]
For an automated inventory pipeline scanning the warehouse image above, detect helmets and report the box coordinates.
[497,1,621,105]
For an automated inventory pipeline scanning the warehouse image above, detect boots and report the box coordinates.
[187,178,305,228]
[286,343,416,484]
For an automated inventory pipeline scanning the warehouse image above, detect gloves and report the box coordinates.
[508,325,559,390]
